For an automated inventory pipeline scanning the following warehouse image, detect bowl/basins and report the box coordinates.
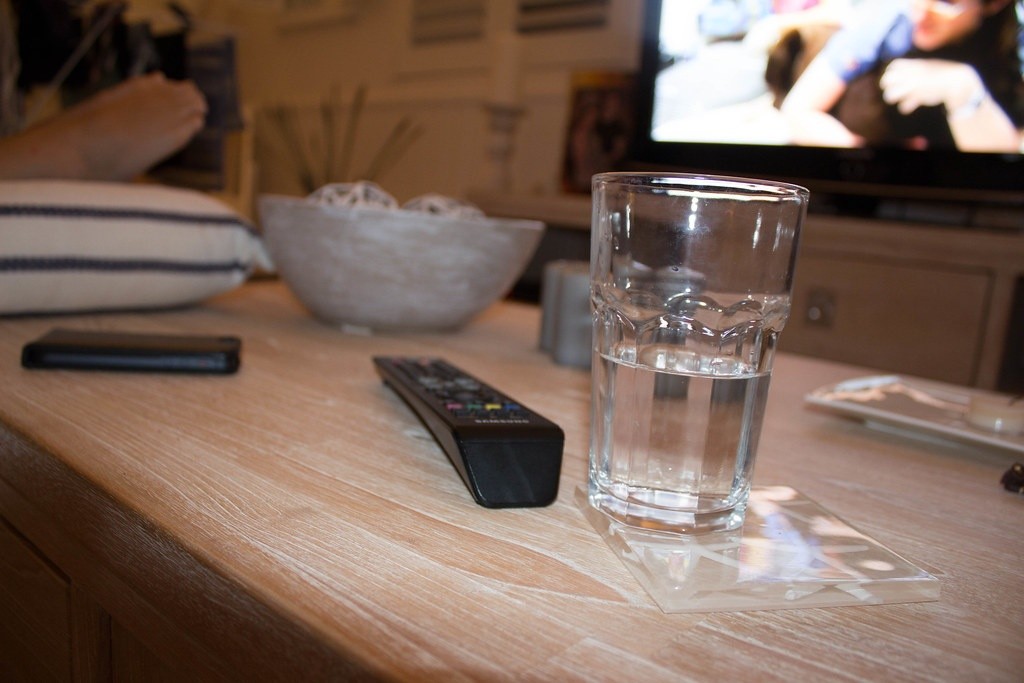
[254,192,547,338]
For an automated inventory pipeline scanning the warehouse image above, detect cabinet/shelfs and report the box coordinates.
[468,187,1024,393]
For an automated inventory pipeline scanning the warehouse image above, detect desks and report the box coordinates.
[0,282,1024,683]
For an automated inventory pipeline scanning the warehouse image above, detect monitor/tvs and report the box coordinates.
[630,0,1024,226]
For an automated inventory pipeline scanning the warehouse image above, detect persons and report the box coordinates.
[0,73,206,181]
[777,0,1024,152]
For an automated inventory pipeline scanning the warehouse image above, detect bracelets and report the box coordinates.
[946,88,985,127]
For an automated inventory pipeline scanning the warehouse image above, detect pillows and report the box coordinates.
[0,178,275,314]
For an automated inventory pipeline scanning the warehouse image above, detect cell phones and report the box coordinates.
[23,329,243,376]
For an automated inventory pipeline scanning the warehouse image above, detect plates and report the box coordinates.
[805,373,1024,467]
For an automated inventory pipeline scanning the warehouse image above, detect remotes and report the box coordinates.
[371,352,564,509]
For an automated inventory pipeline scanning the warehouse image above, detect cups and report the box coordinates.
[584,171,810,541]
